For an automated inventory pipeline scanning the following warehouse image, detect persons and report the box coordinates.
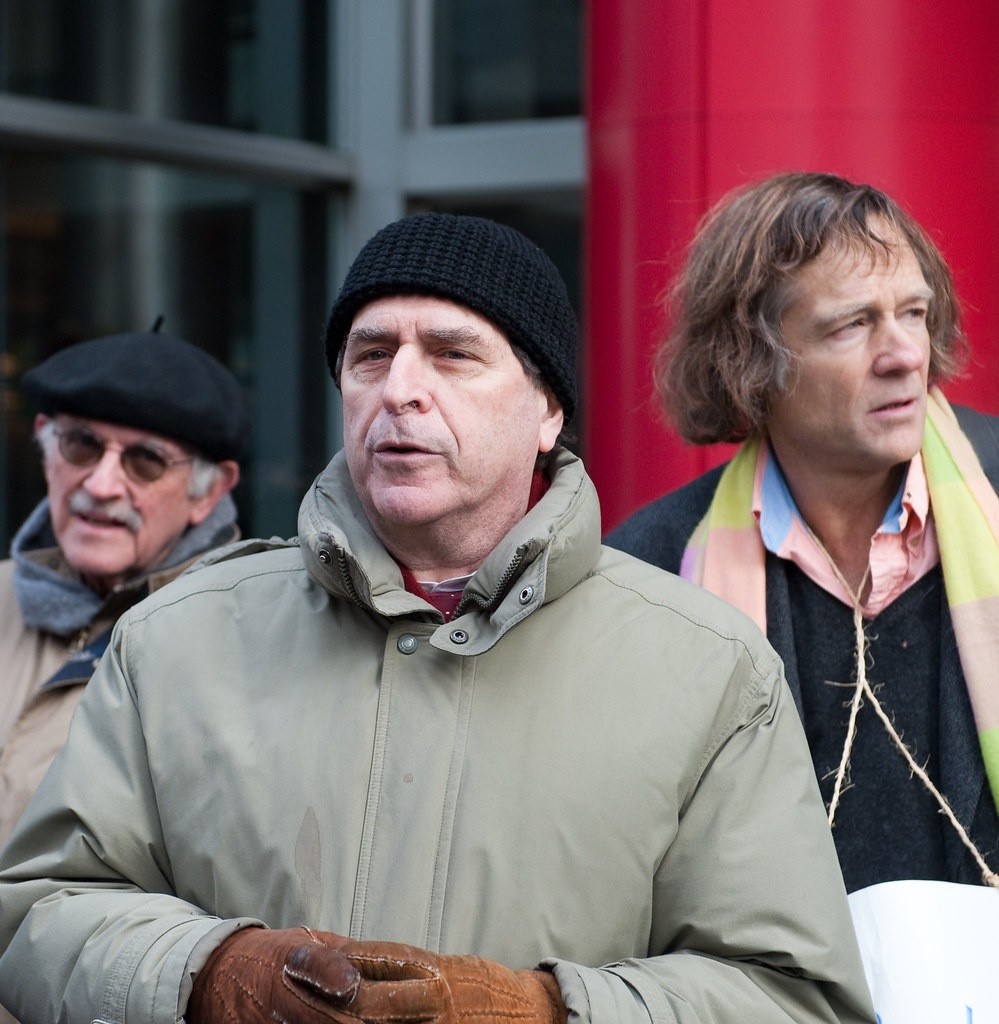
[0,173,999,1024]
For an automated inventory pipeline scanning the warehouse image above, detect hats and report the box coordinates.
[18,313,252,465]
[323,213,582,424]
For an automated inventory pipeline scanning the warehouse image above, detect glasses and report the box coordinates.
[52,424,195,486]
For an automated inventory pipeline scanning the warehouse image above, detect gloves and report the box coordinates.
[184,926,362,1024]
[308,928,568,1024]
